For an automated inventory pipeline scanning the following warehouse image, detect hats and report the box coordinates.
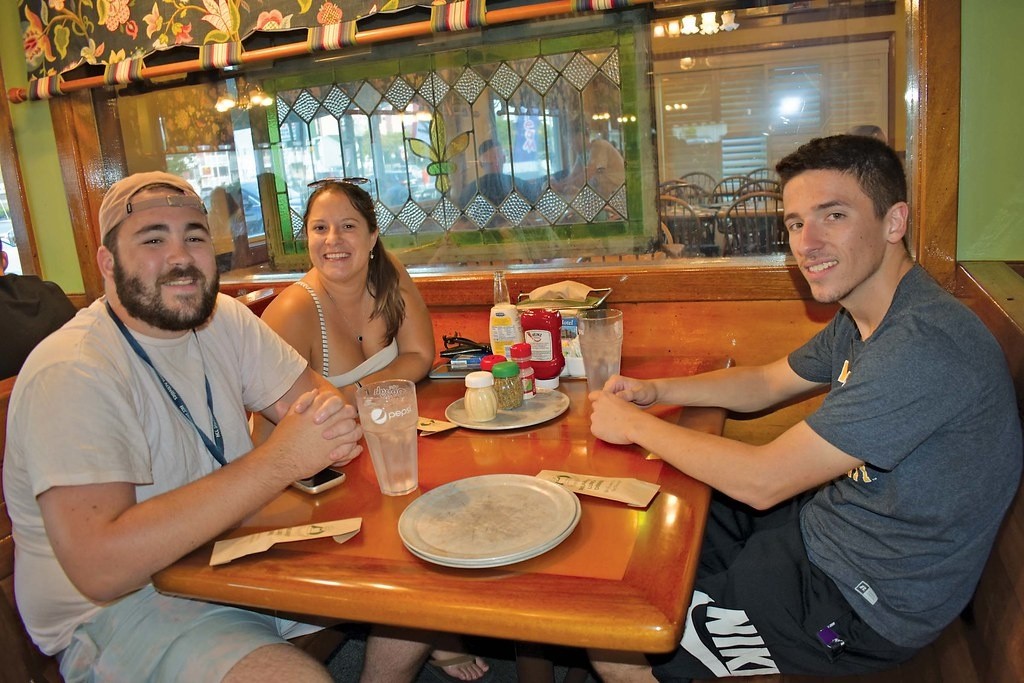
[566,119,591,137]
[98,171,208,246]
[479,140,504,163]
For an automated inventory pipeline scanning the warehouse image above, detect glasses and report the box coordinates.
[870,127,880,138]
[307,177,372,207]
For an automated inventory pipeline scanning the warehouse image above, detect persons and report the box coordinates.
[250,182,492,682]
[1,171,442,682]
[587,133,1024,683]
[458,138,541,225]
[543,116,626,221]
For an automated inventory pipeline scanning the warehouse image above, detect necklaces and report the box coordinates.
[328,294,364,342]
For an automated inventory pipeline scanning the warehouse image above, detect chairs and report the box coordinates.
[456,168,794,267]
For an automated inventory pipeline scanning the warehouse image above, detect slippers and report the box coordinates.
[424,645,495,683]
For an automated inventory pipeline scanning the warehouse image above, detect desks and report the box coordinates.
[150,356,736,683]
[671,190,745,198]
[660,201,784,258]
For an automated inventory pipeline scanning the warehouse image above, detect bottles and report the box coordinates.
[481,355,523,410]
[489,305,523,362]
[493,271,510,307]
[464,371,498,422]
[520,308,565,391]
[510,342,536,400]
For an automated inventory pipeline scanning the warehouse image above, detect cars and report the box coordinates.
[396,165,422,180]
[201,182,302,236]
[508,170,547,180]
[361,172,418,194]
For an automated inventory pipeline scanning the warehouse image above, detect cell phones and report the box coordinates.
[291,468,346,495]
[429,364,481,379]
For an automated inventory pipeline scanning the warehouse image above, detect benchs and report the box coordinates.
[720,261,1024,683]
[0,287,348,683]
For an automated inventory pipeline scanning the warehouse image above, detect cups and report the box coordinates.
[354,379,419,496]
[577,309,623,392]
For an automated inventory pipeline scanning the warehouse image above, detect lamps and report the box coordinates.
[653,10,740,38]
[215,77,272,113]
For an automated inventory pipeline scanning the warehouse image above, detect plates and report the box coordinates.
[445,388,570,430]
[398,473,581,569]
